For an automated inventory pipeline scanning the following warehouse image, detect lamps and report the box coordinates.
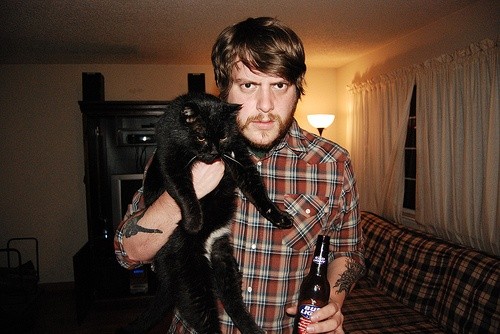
[309,114,336,137]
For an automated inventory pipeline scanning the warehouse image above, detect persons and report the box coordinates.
[114,17,365,334]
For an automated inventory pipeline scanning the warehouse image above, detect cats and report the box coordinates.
[142,90,295,334]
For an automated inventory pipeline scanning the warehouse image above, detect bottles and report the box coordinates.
[293,235,330,334]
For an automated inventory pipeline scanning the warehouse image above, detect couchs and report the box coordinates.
[342,210,500,334]
